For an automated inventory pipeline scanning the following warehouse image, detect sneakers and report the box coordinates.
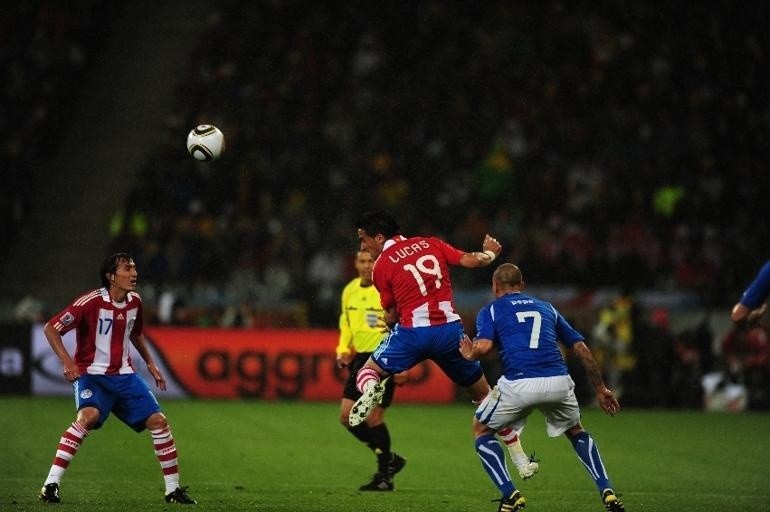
[164,487,196,505]
[390,452,403,478]
[38,483,60,504]
[496,489,525,511]
[517,459,542,480]
[360,478,396,493]
[348,383,384,427]
[601,489,626,512]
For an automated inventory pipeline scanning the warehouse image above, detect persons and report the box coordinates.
[458,263,629,512]
[348,206,540,482]
[40,252,197,505]
[729,259,770,323]
[335,251,408,492]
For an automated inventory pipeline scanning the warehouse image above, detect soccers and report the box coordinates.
[185,123,223,164]
